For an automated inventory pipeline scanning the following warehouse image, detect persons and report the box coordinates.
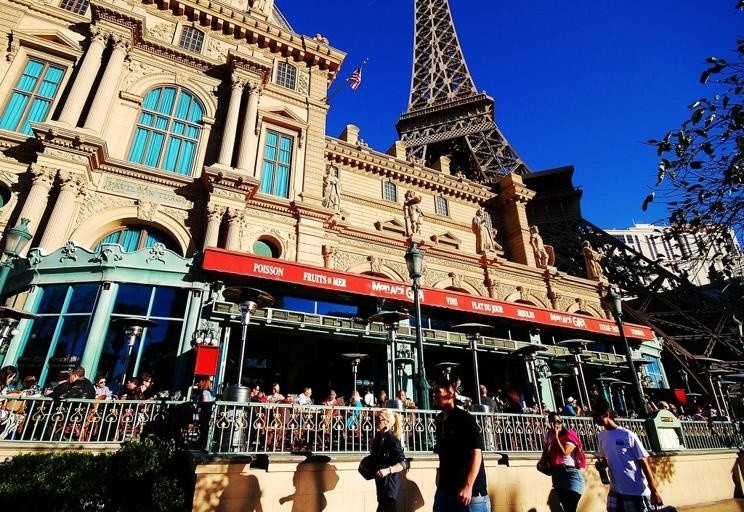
[593,399,677,511]
[324,166,341,213]
[530,224,550,266]
[583,238,605,279]
[359,410,425,512]
[2,361,743,461]
[544,412,587,512]
[732,450,744,511]
[432,385,492,511]
[472,208,495,254]
[405,188,424,232]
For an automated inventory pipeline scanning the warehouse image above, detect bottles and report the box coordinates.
[596,456,610,485]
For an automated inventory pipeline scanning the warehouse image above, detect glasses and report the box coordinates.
[551,420,562,424]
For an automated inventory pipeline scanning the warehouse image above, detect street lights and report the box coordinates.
[222,285,276,383]
[0,305,39,366]
[678,368,692,394]
[112,317,159,400]
[605,286,650,420]
[692,355,738,423]
[451,322,632,412]
[386,356,415,390]
[404,243,436,451]
[434,361,460,381]
[368,310,414,401]
[341,352,369,391]
[617,356,654,386]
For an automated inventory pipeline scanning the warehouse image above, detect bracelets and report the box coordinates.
[389,465,393,474]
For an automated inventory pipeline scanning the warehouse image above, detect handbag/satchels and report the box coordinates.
[537,448,552,476]
[358,454,392,480]
[646,504,677,512]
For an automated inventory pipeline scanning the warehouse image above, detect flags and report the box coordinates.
[350,67,362,92]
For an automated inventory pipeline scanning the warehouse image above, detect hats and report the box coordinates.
[564,396,574,404]
